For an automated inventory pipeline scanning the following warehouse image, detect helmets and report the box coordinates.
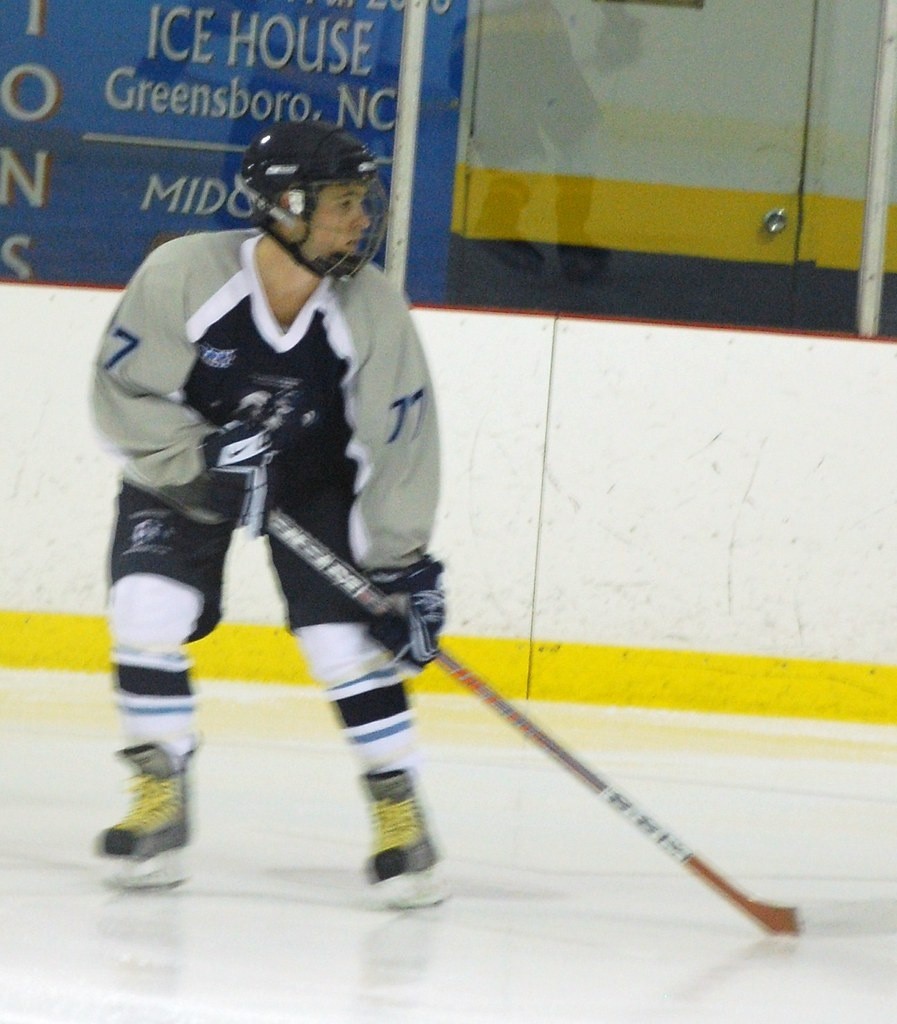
[236,119,390,278]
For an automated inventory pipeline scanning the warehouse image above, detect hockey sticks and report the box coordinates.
[264,507,895,945]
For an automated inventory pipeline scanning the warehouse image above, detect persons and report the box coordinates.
[447,0,649,275]
[90,122,454,911]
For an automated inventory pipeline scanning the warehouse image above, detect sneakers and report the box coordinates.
[364,770,456,909]
[84,742,204,888]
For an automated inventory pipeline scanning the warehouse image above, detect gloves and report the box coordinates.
[200,414,278,525]
[364,554,447,667]
